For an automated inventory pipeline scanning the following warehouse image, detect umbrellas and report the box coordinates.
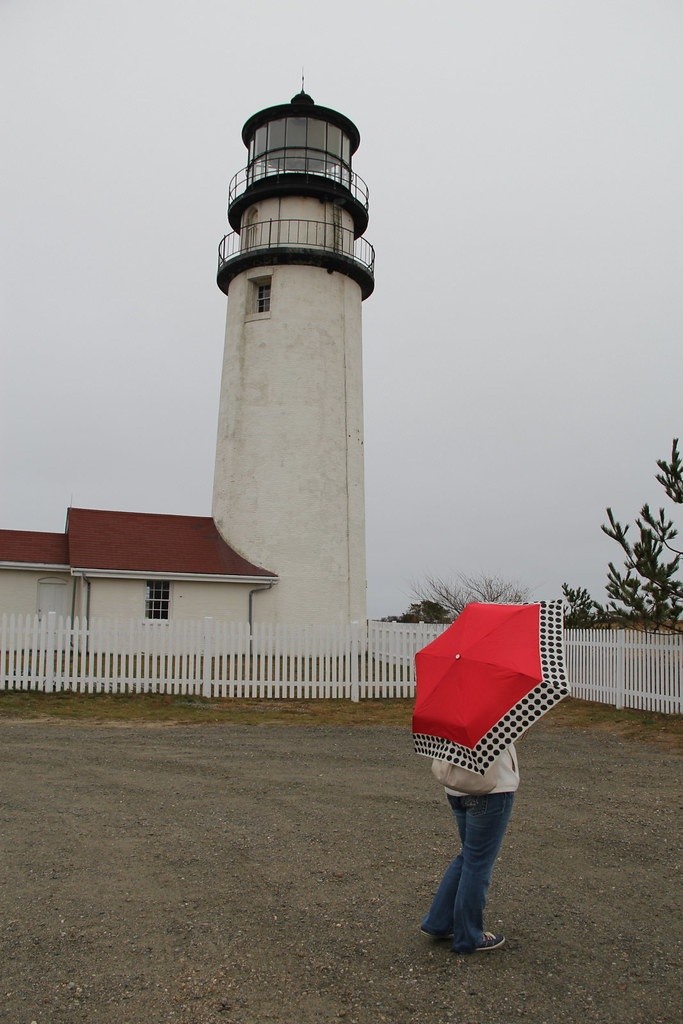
[412,602,574,776]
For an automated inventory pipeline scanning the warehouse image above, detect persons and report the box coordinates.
[419,740,520,953]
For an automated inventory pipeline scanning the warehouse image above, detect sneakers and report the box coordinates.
[474,932,505,950]
[419,928,453,939]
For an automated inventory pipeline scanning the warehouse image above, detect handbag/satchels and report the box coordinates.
[430,758,497,795]
